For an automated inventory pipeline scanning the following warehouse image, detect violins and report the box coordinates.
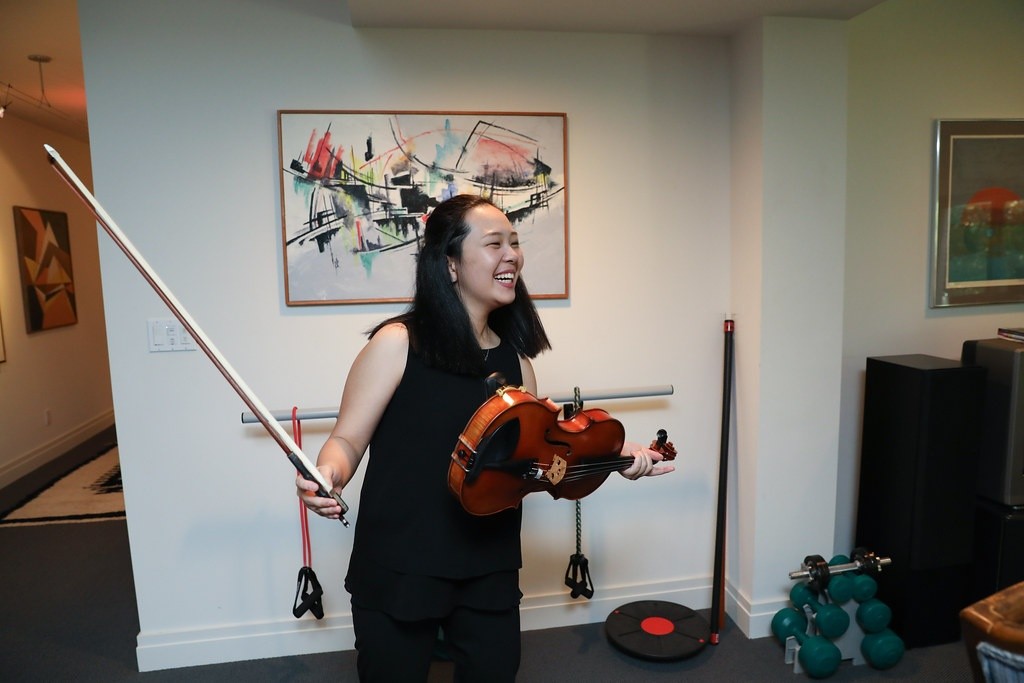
[444,377,681,524]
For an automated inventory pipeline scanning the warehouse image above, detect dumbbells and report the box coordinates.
[771,545,904,683]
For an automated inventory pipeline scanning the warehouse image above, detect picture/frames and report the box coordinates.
[929,119,1024,310]
[277,110,569,307]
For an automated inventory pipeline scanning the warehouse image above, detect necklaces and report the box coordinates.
[484,325,490,363]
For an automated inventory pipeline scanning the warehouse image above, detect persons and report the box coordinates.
[296,193,677,683]
